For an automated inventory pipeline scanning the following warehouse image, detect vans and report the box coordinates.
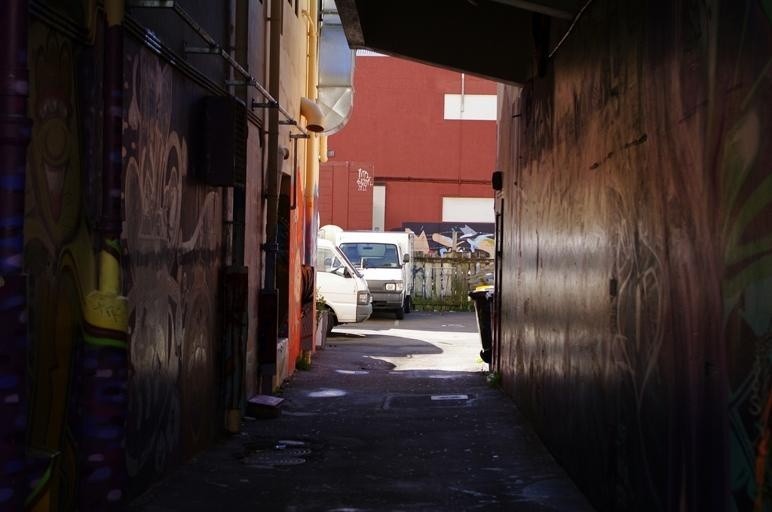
[316,236,372,334]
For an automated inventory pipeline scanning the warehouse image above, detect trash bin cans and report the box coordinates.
[469,291,492,362]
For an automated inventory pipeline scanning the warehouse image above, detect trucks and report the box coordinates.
[324,230,417,320]
